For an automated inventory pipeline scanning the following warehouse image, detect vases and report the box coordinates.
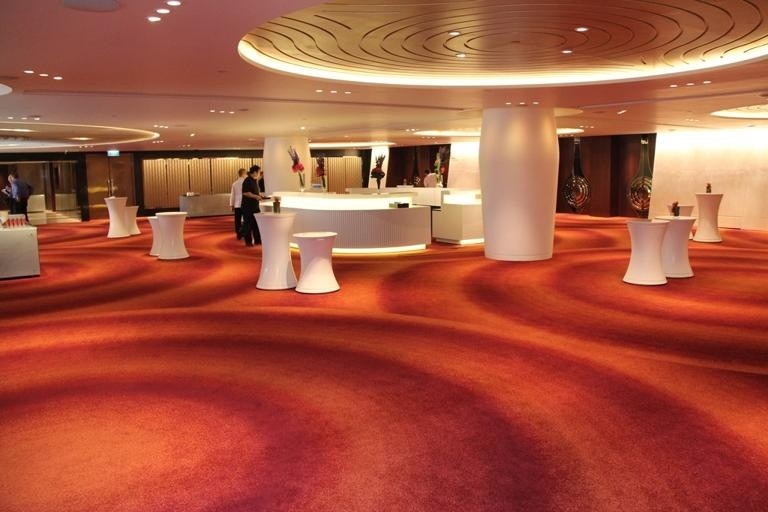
[297,172,306,192]
[320,176,327,192]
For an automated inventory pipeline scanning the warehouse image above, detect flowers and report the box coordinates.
[369,155,386,189]
[287,144,308,187]
[315,153,326,189]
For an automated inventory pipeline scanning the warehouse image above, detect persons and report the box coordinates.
[1,170,34,222]
[229,164,265,247]
[423,166,447,187]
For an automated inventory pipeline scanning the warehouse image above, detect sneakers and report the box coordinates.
[237,231,262,247]
